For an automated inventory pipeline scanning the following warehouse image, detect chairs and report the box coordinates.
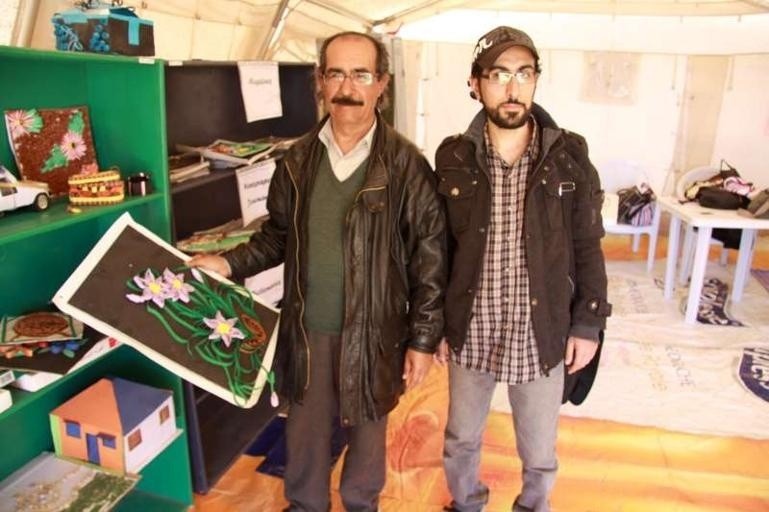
[600,191,660,273]
[677,165,760,290]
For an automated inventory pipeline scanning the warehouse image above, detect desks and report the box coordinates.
[657,195,769,325]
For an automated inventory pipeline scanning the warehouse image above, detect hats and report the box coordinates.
[472,27,537,74]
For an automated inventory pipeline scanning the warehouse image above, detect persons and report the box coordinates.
[183,33,450,512]
[430,26,612,511]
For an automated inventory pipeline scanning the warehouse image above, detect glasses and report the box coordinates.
[324,70,376,88]
[483,72,535,85]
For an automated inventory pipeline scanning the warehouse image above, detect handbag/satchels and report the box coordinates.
[563,332,604,407]
[616,184,656,226]
[696,160,746,209]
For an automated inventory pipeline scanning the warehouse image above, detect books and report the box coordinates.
[167,135,300,183]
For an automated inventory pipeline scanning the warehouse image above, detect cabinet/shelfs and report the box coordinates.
[1,45,194,510]
[163,60,322,496]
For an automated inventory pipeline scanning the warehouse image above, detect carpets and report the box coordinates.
[489,250,769,441]
